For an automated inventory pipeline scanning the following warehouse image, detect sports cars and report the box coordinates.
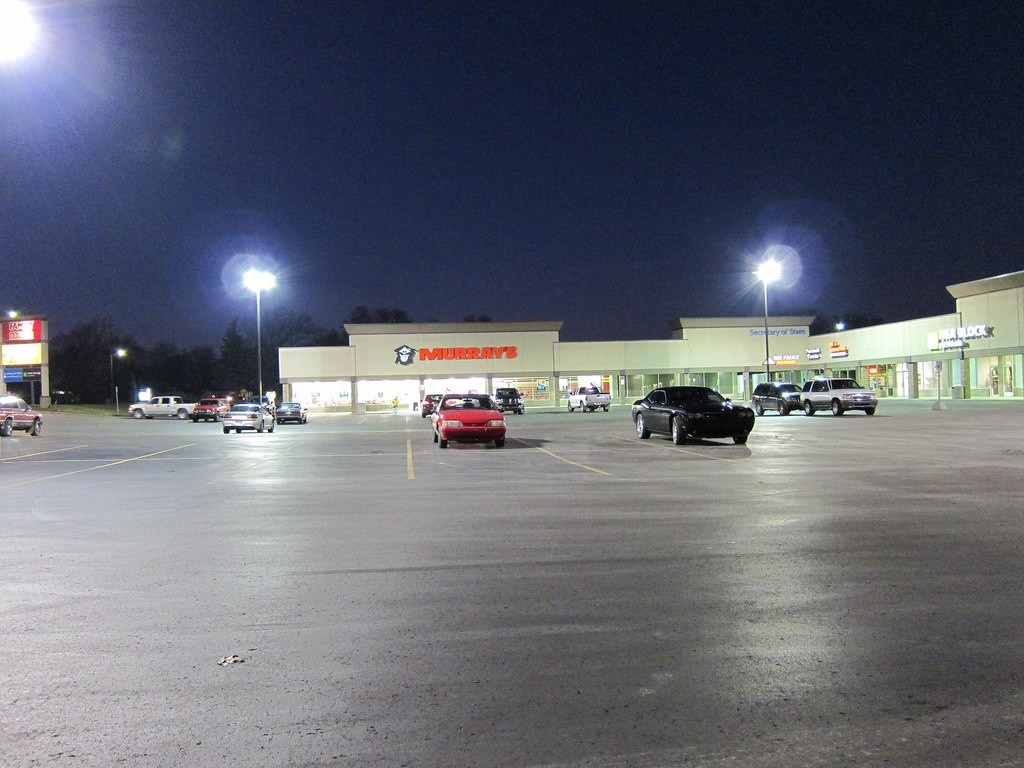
[631,385,756,445]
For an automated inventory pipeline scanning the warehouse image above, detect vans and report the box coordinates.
[248,394,269,411]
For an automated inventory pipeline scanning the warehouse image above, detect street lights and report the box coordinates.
[247,271,275,404]
[759,266,774,382]
[109,349,125,395]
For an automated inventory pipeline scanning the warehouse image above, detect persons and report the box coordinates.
[269,400,275,418]
[392,396,399,413]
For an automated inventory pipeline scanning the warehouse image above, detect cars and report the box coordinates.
[429,393,506,448]
[0,396,43,437]
[275,402,308,424]
[222,403,275,434]
[421,394,443,418]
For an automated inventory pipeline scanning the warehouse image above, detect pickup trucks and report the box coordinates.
[568,386,611,412]
[128,396,200,419]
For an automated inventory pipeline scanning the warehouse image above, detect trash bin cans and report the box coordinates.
[413,402,418,411]
[951,385,964,399]
[888,388,893,396]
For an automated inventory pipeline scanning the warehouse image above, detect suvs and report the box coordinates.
[800,377,878,417]
[752,383,806,416]
[194,398,230,422]
[491,387,526,415]
